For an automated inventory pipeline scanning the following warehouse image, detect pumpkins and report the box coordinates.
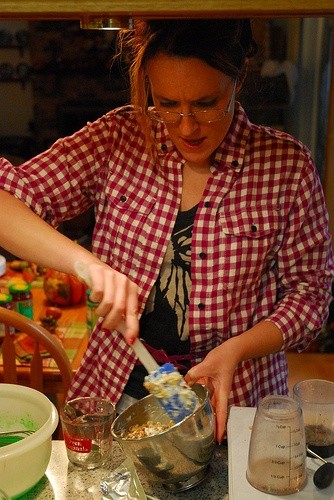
[42,270,88,305]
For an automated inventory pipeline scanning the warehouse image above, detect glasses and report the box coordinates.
[143,76,238,123]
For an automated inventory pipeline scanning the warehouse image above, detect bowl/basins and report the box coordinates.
[0,255,7,276]
[0,383,59,500]
[110,382,215,493]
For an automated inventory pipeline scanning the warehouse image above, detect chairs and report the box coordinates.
[0,307,73,440]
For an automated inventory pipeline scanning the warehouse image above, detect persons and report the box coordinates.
[0,19,334,444]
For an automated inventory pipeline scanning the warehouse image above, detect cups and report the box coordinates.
[245,395,308,495]
[293,378,334,458]
[60,397,116,470]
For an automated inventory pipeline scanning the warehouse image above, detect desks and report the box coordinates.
[0,263,90,440]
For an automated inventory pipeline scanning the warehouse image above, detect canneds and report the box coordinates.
[8,284,34,332]
[85,288,101,334]
[0,293,15,346]
